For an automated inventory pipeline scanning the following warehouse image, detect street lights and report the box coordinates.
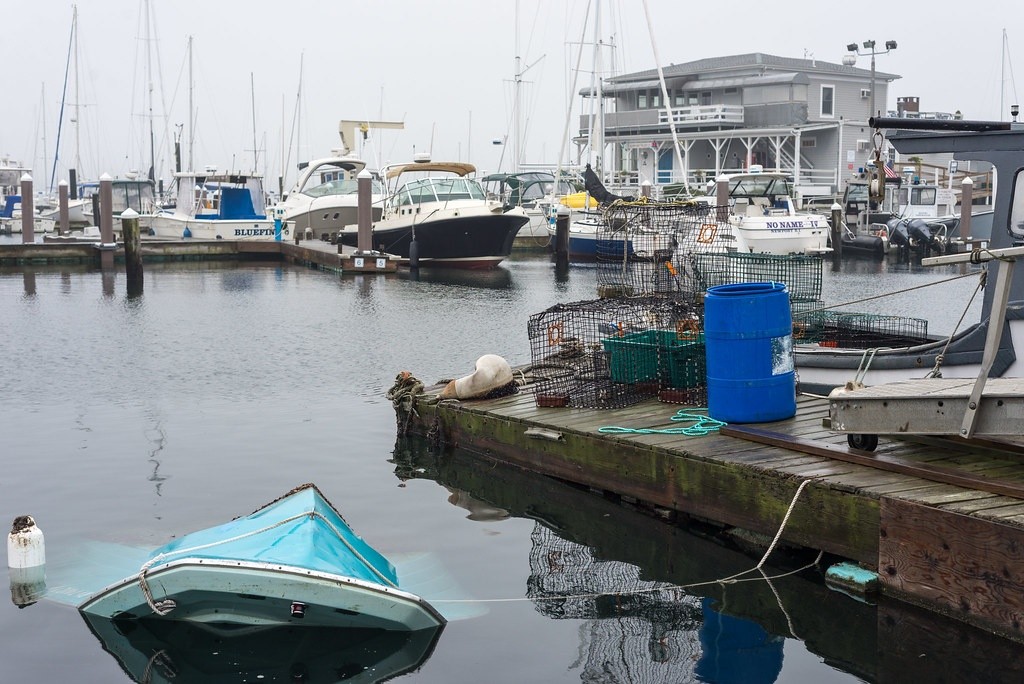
[846,39,898,158]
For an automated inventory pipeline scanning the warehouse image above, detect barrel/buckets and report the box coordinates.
[693,598,785,684]
[704,281,798,423]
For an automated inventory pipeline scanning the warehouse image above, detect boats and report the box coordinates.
[0,0,840,273]
[792,29,1024,394]
[76,482,450,637]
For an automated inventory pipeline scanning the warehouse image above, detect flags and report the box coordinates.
[884,160,897,178]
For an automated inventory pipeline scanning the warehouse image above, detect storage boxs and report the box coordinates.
[538,394,570,408]
[602,328,707,391]
